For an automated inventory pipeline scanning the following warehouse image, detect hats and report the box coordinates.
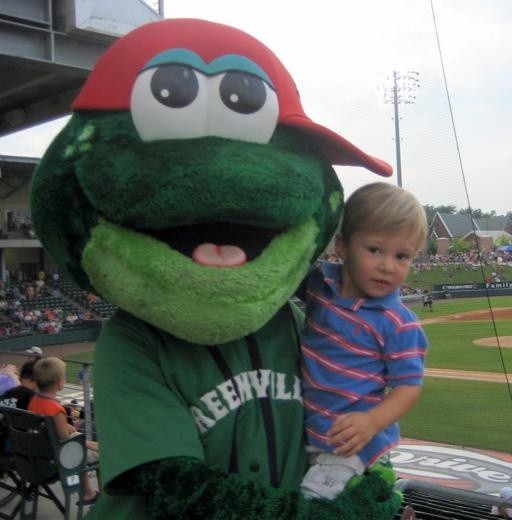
[26,346,43,354]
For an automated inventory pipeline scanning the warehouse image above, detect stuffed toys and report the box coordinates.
[26,18,416,519]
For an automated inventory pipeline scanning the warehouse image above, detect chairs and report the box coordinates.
[0,398,99,519]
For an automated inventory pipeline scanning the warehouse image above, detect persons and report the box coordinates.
[402,246,512,310]
[296,182,428,502]
[0,216,100,505]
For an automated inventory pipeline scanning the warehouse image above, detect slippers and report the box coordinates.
[76,488,100,505]
